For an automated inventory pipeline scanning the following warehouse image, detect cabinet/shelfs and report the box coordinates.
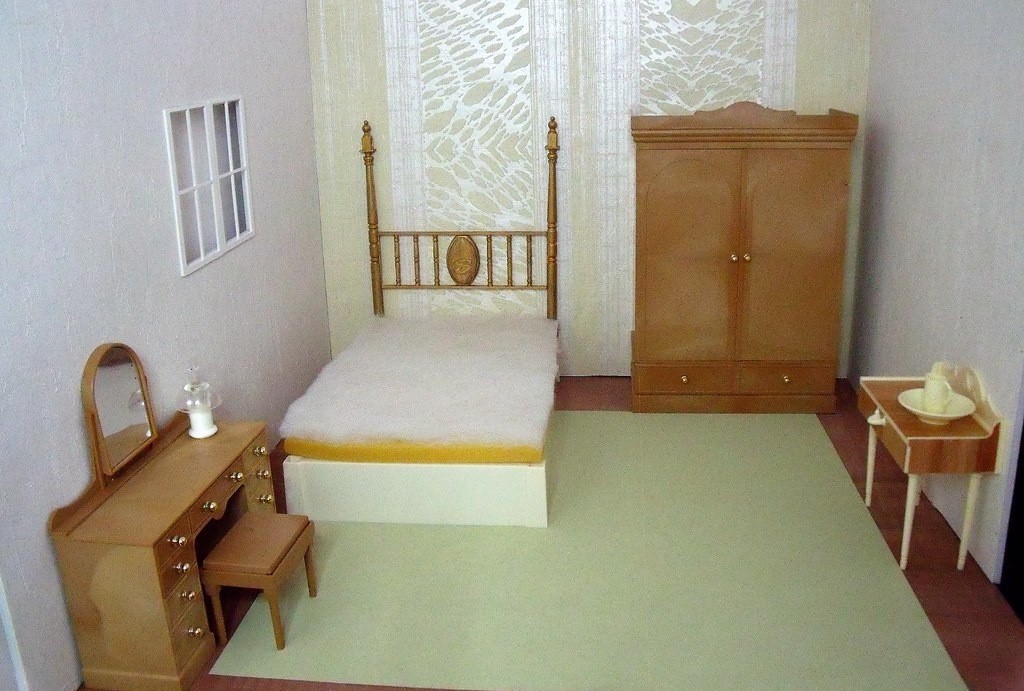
[631,100,858,414]
[50,410,277,691]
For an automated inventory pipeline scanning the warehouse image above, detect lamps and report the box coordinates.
[174,361,222,438]
[130,390,151,436]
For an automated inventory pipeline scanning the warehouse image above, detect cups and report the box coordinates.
[922,373,953,415]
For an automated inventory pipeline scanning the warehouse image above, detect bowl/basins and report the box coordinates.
[898,388,976,426]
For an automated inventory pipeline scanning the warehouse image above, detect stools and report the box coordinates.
[200,512,317,651]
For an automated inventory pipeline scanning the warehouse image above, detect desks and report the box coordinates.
[857,361,1001,571]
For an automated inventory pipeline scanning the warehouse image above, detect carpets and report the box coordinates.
[208,411,971,691]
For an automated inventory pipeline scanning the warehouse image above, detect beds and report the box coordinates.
[280,116,560,527]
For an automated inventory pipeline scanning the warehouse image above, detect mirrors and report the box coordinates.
[81,342,159,476]
